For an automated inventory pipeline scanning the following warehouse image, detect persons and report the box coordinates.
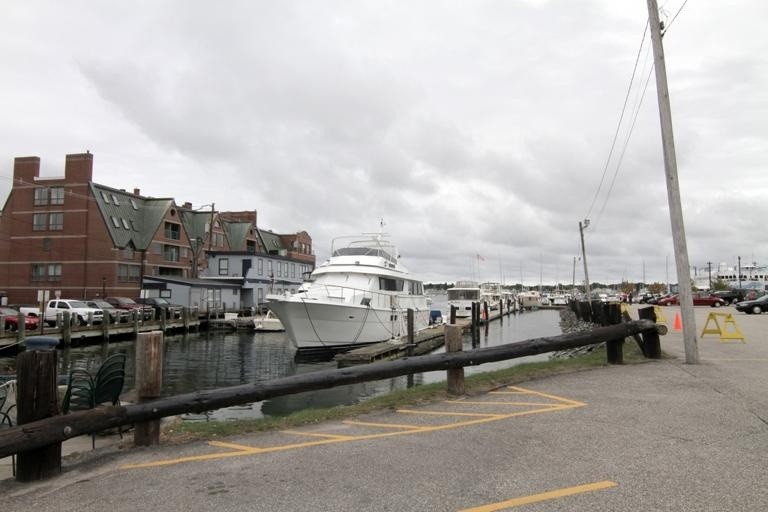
[620,291,627,304]
[627,293,632,305]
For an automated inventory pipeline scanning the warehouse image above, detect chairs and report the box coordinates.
[0,352,126,477]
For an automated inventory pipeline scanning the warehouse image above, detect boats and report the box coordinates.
[447,281,571,317]
[252,217,432,349]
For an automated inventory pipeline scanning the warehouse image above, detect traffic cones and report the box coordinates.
[672,310,682,330]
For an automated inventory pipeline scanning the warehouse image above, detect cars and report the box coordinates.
[0,296,184,332]
[599,289,767,314]
